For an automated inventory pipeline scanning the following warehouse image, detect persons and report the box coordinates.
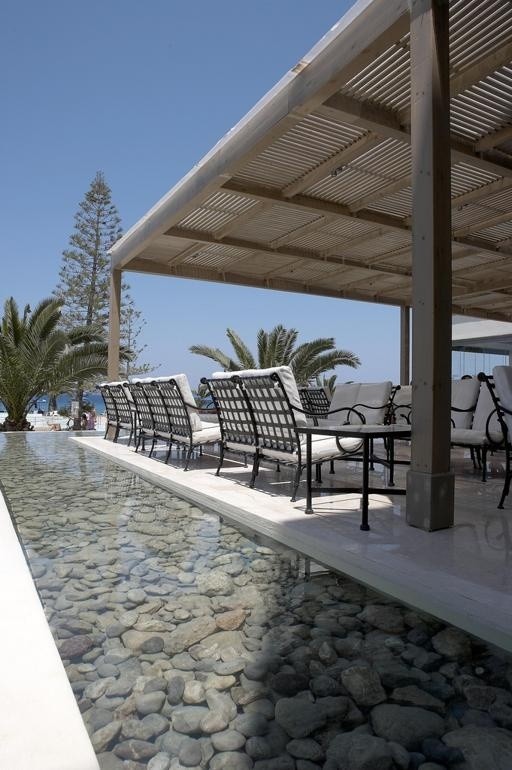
[81,406,97,430]
[47,423,61,431]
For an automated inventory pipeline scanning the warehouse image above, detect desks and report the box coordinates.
[294,423,412,530]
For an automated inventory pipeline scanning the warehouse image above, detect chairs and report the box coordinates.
[96,364,512,510]
